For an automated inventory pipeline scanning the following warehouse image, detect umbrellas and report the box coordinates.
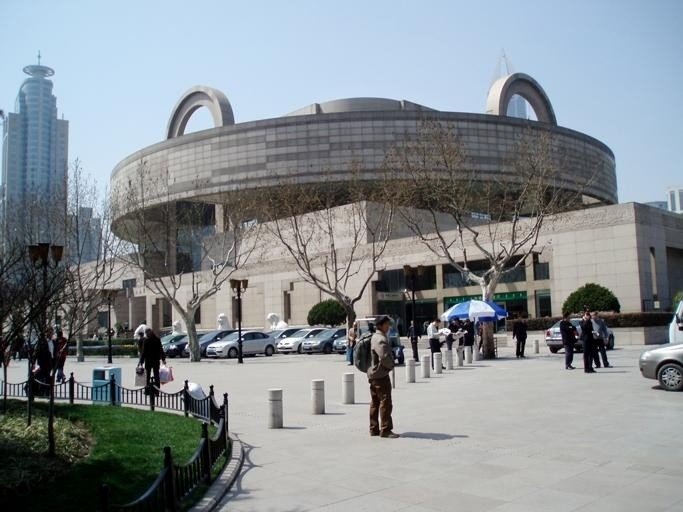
[440,299,508,344]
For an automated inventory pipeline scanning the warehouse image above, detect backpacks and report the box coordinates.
[352,332,373,374]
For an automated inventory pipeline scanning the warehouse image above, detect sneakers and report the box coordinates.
[368,430,400,439]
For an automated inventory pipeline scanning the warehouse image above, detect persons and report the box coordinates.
[348,312,610,373]
[131,319,169,396]
[0,321,70,397]
[366,316,400,437]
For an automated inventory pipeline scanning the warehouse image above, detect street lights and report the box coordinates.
[24,240,67,466]
[105,293,114,364]
[400,262,426,363]
[228,277,249,364]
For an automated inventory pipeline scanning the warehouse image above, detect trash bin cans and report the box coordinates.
[92,367,121,405]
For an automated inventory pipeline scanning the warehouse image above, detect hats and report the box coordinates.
[373,315,391,327]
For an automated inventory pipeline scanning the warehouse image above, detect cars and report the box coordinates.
[637,339,682,393]
[154,325,371,361]
[543,316,614,354]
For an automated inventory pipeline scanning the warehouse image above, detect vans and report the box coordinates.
[667,298,682,345]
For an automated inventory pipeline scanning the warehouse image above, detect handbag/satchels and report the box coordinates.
[135,364,147,387]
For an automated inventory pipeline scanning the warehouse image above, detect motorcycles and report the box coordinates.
[347,313,406,365]
